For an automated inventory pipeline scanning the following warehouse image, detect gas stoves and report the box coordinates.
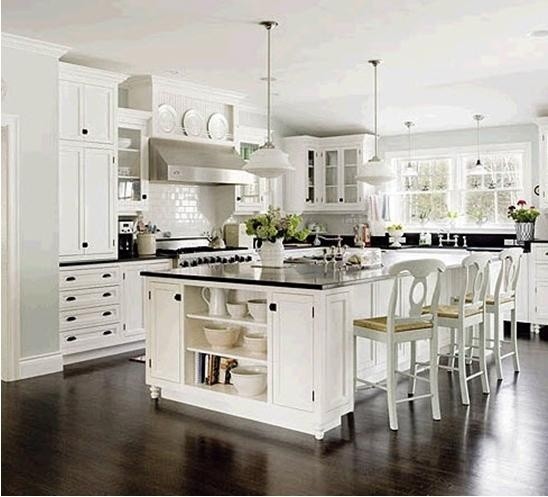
[157,245,251,266]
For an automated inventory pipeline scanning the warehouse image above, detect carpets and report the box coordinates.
[128,351,145,363]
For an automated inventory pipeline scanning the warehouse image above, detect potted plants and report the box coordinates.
[386,224,407,248]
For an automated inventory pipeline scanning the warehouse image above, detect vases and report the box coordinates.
[253,236,285,268]
[515,222,533,243]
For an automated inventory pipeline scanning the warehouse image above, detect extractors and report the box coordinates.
[148,137,254,186]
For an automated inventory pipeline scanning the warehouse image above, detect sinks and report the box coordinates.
[395,248,467,254]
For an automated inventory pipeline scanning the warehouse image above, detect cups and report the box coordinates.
[307,223,315,230]
[136,233,156,256]
[314,227,320,232]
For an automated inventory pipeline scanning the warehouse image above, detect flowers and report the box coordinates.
[506,201,541,222]
[244,205,312,240]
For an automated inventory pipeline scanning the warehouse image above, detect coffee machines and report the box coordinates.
[118,219,134,259]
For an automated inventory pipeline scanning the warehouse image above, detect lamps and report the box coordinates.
[241,21,296,178]
[469,114,486,174]
[402,122,419,178]
[355,57,396,186]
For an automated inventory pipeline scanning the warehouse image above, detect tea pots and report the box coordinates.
[205,224,225,249]
[201,287,229,317]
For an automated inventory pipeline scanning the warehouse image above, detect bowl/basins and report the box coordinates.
[201,323,240,352]
[226,301,249,319]
[248,298,267,322]
[228,365,267,397]
[243,332,267,355]
[118,166,132,176]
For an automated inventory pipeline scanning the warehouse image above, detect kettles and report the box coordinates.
[354,224,370,246]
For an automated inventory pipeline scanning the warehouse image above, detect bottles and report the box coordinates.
[424,230,431,244]
[419,230,424,240]
[312,233,320,245]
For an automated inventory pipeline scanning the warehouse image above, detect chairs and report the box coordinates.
[465,245,523,382]
[409,251,492,406]
[349,259,450,430]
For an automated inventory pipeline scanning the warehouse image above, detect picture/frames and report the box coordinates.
[118,108,152,217]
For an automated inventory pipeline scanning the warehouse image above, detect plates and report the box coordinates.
[206,112,229,140]
[182,108,205,138]
[118,137,131,148]
[158,103,178,136]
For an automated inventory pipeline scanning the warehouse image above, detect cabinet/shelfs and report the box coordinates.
[143,276,355,441]
[121,259,173,353]
[281,134,321,214]
[533,116,548,209]
[59,262,121,366]
[237,131,281,213]
[320,133,373,215]
[59,62,131,263]
[529,242,548,335]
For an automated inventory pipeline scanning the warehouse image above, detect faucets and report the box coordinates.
[446,229,450,240]
[463,236,466,246]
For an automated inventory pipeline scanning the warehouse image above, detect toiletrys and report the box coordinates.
[418,230,426,246]
[425,231,432,246]
